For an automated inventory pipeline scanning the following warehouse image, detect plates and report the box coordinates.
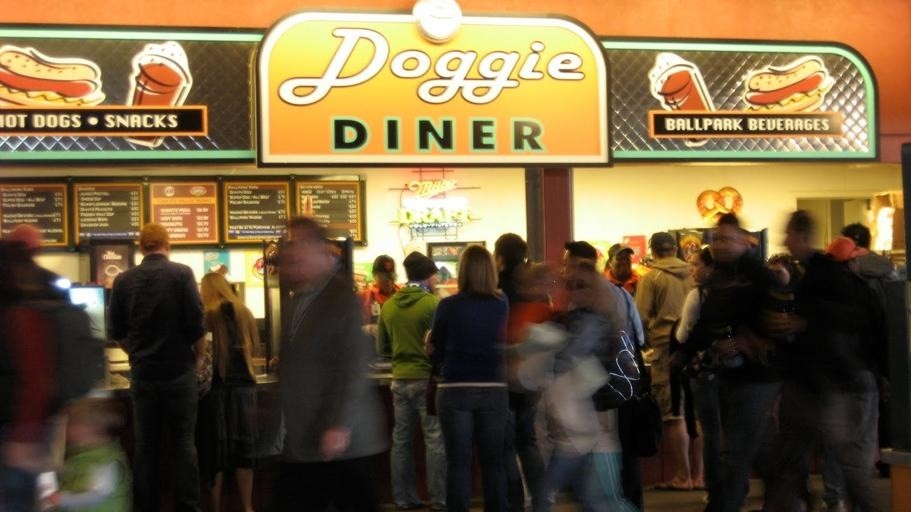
[366,360,392,373]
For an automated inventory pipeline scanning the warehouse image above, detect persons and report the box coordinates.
[635,210,911,511]
[2,209,644,512]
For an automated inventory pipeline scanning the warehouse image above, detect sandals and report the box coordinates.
[652,477,708,492]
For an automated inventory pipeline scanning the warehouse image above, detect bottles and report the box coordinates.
[720,325,744,369]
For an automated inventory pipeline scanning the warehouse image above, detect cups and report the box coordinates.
[123,53,191,148]
[35,470,58,498]
[654,63,716,148]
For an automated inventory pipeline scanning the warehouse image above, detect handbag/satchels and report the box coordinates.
[590,325,646,414]
[616,389,666,462]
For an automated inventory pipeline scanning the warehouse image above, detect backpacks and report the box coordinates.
[20,296,101,402]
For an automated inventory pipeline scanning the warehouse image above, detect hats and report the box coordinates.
[139,221,170,253]
[824,235,872,263]
[0,223,43,249]
[401,249,442,284]
[371,255,399,281]
[606,243,636,258]
[648,232,679,254]
